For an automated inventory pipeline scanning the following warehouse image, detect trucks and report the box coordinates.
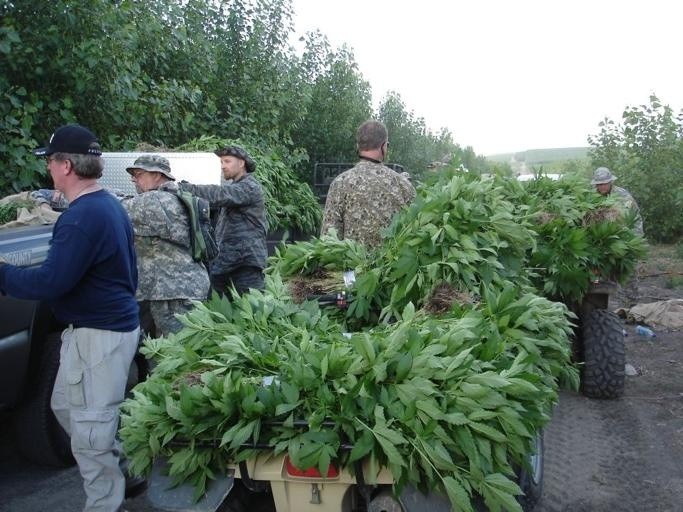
[311,161,408,214]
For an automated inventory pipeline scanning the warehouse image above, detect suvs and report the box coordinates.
[419,205,623,399]
[143,273,544,511]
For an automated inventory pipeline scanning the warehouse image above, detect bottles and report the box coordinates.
[634,325,657,337]
[623,329,628,338]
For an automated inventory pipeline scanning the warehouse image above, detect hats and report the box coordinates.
[126,153,176,182]
[33,124,102,158]
[215,145,257,173]
[591,166,619,187]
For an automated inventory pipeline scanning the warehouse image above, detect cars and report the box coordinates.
[516,172,566,186]
[0,209,299,471]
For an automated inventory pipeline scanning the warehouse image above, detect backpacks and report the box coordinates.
[154,187,219,263]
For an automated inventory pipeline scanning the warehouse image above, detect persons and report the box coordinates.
[590,168,644,319]
[51,152,214,340]
[0,123,148,511]
[176,145,271,302]
[318,118,416,253]
